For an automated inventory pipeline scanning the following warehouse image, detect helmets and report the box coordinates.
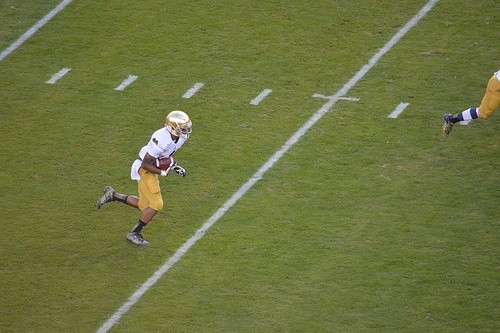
[166,110,192,138]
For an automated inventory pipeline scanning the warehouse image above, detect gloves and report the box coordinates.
[161,163,176,176]
[171,165,186,177]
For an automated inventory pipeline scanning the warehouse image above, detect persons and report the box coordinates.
[443,68,500,136]
[96,110,192,246]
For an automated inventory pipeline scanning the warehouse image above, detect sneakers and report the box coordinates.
[96,186,115,209]
[442,113,453,136]
[124,232,149,246]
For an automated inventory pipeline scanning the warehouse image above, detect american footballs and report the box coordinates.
[153,157,177,170]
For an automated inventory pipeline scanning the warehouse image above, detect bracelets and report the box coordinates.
[171,164,176,170]
[160,169,168,177]
